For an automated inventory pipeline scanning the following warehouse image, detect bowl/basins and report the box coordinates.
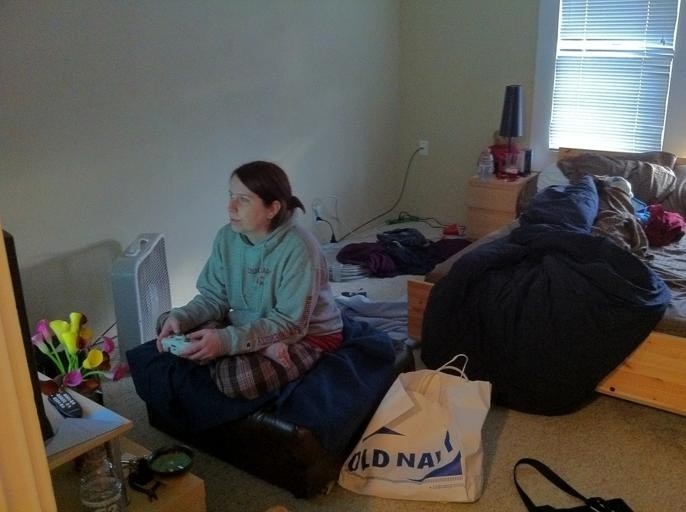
[146,444,195,477]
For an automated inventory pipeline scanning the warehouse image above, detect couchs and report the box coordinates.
[121,312,414,504]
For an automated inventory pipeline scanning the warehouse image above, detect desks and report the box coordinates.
[37,370,212,511]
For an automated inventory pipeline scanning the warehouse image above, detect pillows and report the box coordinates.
[512,150,686,261]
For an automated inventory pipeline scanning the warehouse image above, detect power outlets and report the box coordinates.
[418,140,428,156]
[312,206,324,224]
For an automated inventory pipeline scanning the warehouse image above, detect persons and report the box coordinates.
[144,162,341,451]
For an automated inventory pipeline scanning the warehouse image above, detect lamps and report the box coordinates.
[497,84,525,183]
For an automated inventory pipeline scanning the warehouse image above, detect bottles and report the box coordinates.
[78,445,124,512]
[477,147,493,184]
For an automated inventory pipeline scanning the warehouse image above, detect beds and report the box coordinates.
[406,216,686,418]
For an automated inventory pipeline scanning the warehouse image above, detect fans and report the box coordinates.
[109,230,178,359]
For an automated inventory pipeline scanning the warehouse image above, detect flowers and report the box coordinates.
[30,309,129,398]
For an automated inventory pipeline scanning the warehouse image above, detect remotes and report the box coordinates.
[48,390,82,419]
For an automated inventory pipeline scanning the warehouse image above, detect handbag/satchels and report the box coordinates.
[512,458,635,512]
[337,352,492,504]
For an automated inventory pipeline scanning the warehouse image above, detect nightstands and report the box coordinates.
[464,171,539,242]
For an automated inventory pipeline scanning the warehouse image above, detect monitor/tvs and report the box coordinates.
[3,228,54,442]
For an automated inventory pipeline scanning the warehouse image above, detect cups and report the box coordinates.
[503,152,519,171]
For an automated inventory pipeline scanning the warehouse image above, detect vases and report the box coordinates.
[67,390,106,469]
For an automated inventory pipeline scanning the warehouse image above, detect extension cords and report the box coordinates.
[386,216,419,225]
